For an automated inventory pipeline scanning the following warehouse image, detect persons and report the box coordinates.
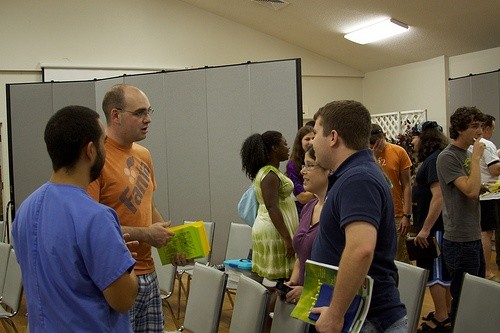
[12,104,139,333]
[241,99,500,333]
[87,83,194,333]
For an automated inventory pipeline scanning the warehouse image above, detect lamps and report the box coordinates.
[343,18,409,46]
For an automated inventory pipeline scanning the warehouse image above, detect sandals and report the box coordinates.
[417,317,452,333]
[422,310,450,320]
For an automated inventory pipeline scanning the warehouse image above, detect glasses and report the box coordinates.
[116,107,154,119]
[302,164,322,171]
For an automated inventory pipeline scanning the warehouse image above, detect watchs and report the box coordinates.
[403,214,411,219]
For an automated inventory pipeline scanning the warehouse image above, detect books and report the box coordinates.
[292,258,374,333]
[156,221,211,266]
[308,285,365,333]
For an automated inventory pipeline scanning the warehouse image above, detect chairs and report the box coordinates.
[0,219,500,333]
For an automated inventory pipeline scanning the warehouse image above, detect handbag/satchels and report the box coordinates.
[238,184,259,227]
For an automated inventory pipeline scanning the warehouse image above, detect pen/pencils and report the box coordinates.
[473,137,477,142]
